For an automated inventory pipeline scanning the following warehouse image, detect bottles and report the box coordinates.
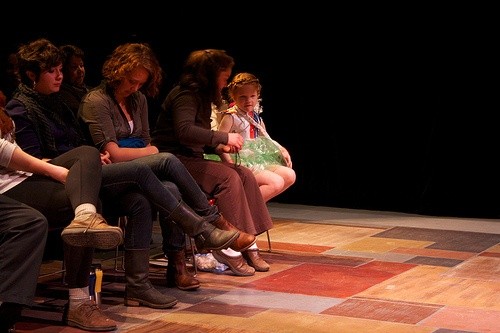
[89,263,102,307]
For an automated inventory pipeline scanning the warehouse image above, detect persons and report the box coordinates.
[4,38,241,333]
[75,42,257,290]
[0,195,48,333]
[3,45,24,104]
[216,72,296,203]
[57,45,95,119]
[150,49,275,276]
[0,90,124,331]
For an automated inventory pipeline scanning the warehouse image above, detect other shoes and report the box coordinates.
[167,250,201,290]
[211,211,257,251]
[212,246,271,276]
[61,213,124,250]
[62,297,117,331]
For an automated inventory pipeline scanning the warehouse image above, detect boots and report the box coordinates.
[165,200,240,252]
[122,248,178,309]
